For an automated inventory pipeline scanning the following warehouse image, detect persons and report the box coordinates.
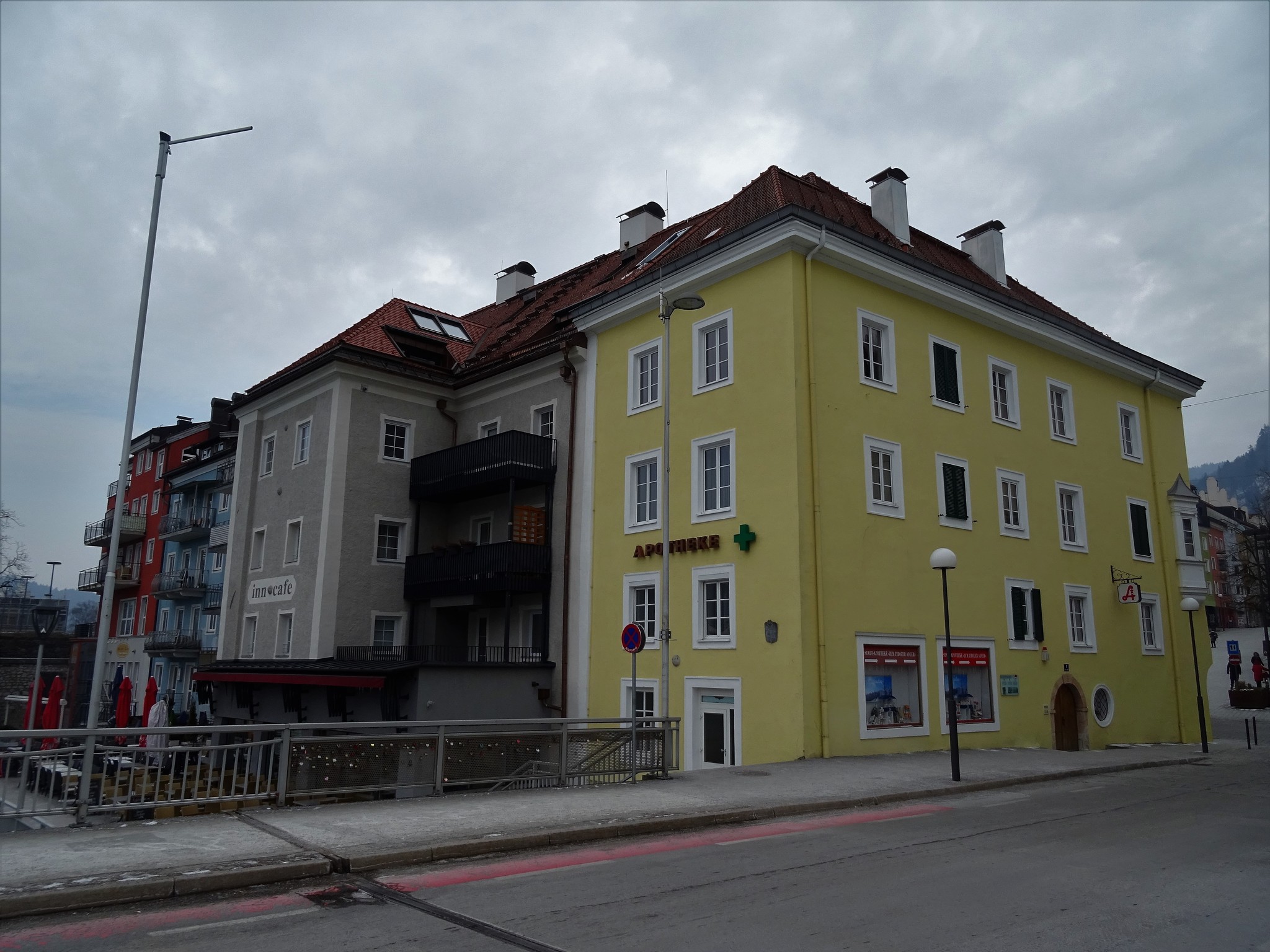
[1251,652,1265,666]
[1209,629,1218,648]
[1227,662,1241,689]
[1251,660,1269,688]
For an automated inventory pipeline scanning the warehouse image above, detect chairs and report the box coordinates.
[185,518,203,527]
[17,754,273,822]
[120,565,132,580]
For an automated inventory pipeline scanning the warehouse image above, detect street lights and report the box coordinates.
[1218,594,1225,631]
[1179,598,1209,754]
[656,290,708,776]
[929,547,961,781]
[16,606,63,810]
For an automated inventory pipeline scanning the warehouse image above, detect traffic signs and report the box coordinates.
[621,624,641,653]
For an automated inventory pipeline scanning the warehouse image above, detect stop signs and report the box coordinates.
[1228,655,1240,665]
